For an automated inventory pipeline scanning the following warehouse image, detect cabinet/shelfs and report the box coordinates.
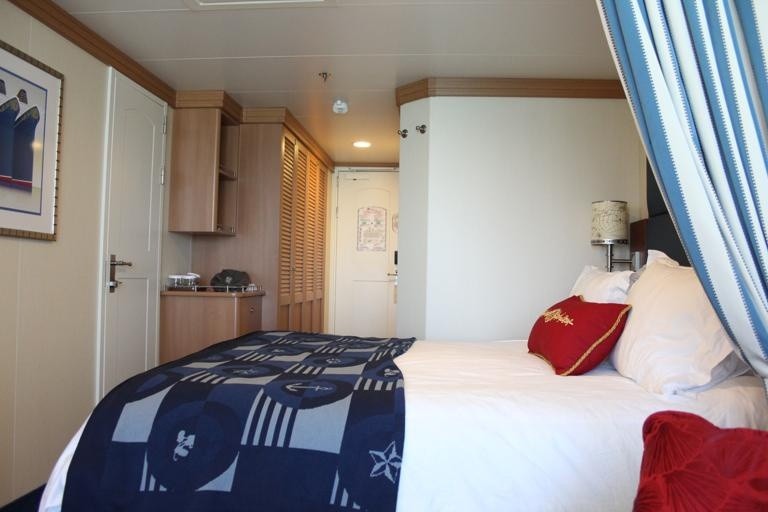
[190,123,330,334]
[172,109,243,236]
[160,296,261,366]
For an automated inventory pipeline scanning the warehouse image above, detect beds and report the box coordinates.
[58,323,768,512]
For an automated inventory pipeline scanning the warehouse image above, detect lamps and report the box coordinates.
[589,199,640,273]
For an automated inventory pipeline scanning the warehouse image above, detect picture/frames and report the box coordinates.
[0,37,67,244]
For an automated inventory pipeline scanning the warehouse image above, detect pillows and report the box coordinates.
[630,411,768,511]
[526,248,751,401]
